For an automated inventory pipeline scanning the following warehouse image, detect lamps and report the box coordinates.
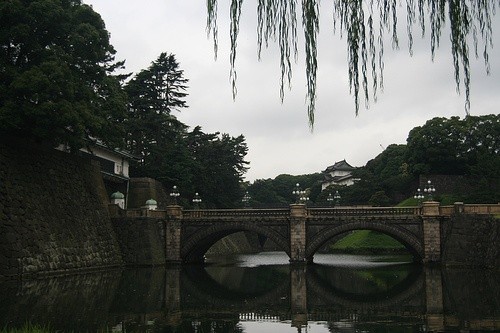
[334,190,342,206]
[327,193,334,208]
[413,188,424,206]
[242,195,248,207]
[299,191,309,203]
[192,193,202,209]
[170,185,180,204]
[424,180,436,201]
[243,191,251,207]
[291,182,304,204]
